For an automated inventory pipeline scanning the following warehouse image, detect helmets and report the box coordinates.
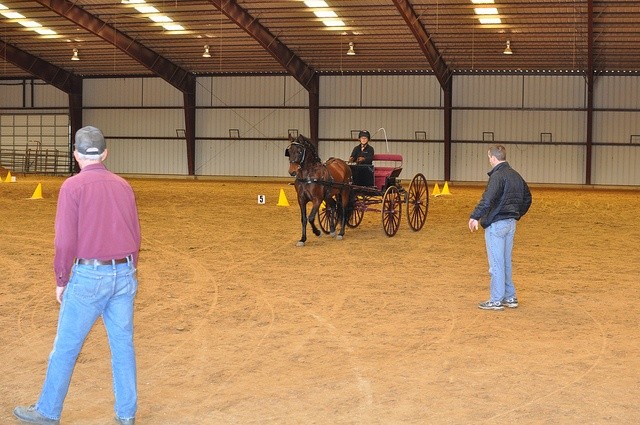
[359,130,370,140]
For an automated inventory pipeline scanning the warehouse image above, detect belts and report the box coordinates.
[74,255,134,266]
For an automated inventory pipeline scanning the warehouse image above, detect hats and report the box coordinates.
[75,126,107,155]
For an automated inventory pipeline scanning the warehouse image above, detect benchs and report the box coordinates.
[372,153,403,194]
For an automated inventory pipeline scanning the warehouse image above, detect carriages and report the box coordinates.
[284,129,429,248]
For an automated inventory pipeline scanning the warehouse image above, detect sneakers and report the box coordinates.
[503,299,518,308]
[478,298,504,310]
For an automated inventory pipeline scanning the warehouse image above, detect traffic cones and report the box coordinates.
[4,170,13,182]
[441,181,451,196]
[30,183,44,199]
[319,198,327,211]
[433,182,442,197]
[277,187,288,207]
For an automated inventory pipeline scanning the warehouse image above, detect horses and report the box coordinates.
[286,133,353,247]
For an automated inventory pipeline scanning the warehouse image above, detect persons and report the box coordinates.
[12,126,142,425]
[348,129,374,165]
[468,144,533,310]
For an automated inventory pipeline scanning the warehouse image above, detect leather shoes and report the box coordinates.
[115,417,135,425]
[14,404,60,425]
